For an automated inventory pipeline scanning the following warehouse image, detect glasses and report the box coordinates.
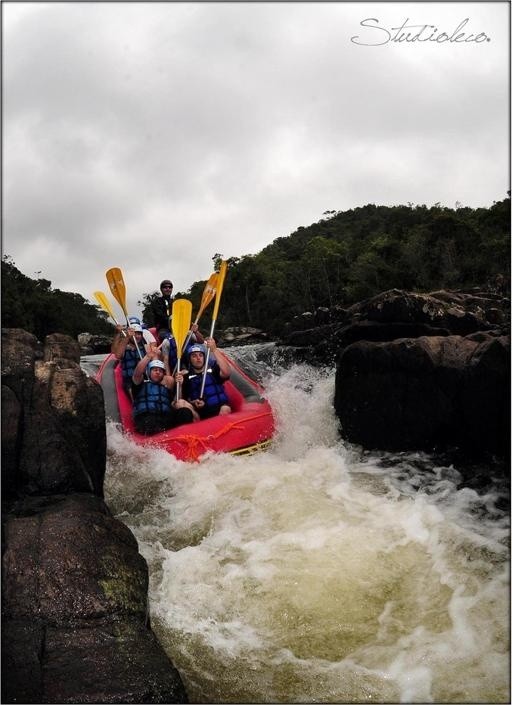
[163,286,173,290]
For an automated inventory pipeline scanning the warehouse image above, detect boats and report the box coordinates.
[95,327,275,464]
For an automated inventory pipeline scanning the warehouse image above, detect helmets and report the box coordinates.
[160,280,173,289]
[130,324,143,334]
[127,317,140,327]
[168,315,172,330]
[147,360,166,378]
[188,344,205,360]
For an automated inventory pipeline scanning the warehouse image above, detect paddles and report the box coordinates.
[201,261,227,400]
[106,268,152,384]
[172,274,218,376]
[172,299,192,403]
[94,292,127,338]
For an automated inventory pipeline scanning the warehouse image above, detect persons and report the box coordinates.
[111,280,232,436]
